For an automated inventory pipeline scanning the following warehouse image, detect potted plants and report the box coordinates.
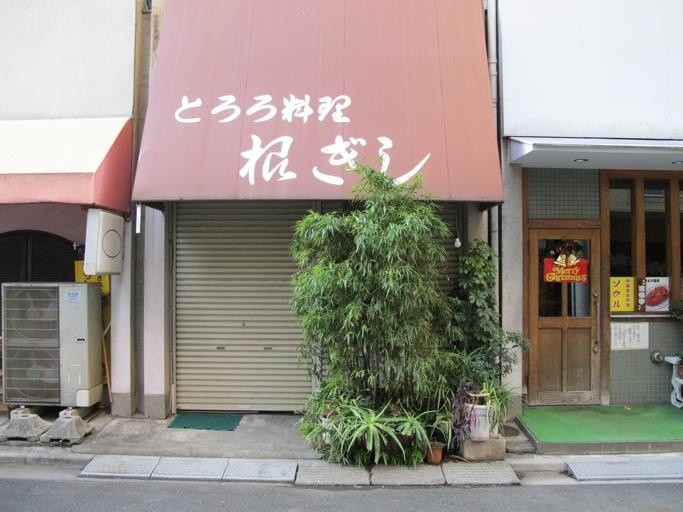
[464,376,505,442]
[673,351,683,378]
[320,399,340,445]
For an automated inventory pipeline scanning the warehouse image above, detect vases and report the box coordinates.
[426,442,443,465]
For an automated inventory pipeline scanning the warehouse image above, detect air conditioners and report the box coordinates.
[0,282,104,408]
